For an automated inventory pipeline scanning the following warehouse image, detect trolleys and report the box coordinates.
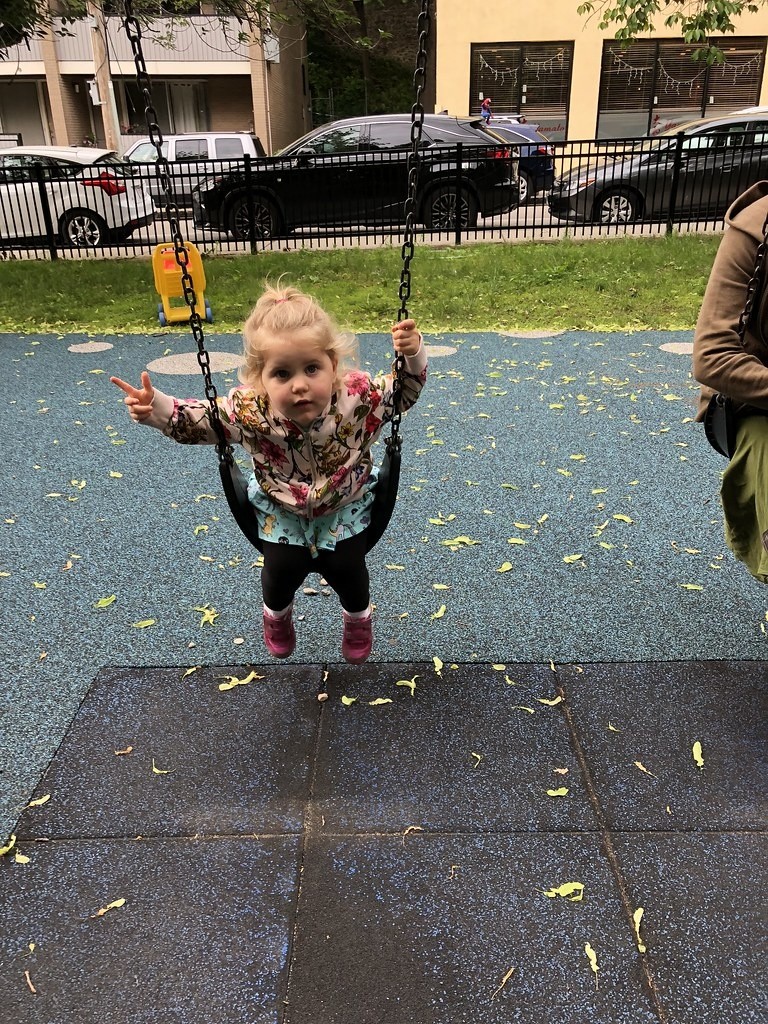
[152,241,212,325]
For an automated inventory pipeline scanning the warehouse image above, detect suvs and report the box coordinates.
[485,124,556,205]
[0,146,157,248]
[117,132,266,211]
[473,115,526,125]
[192,109,518,239]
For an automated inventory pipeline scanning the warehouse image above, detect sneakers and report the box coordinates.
[262,599,296,658]
[342,611,372,664]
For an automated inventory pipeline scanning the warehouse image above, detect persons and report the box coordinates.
[480,98,493,125]
[108,284,430,664]
[688,181,768,593]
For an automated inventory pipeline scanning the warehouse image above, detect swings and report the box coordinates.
[122,0,435,563]
[703,214,768,461]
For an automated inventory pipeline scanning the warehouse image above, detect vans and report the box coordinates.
[545,113,768,221]
